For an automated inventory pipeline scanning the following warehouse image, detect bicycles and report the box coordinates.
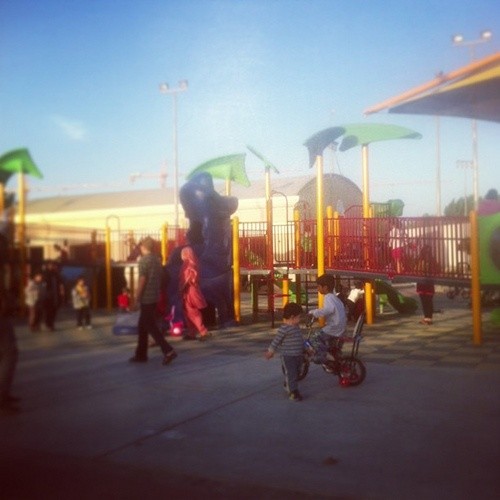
[281,312,366,387]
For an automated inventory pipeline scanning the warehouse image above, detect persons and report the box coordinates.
[416,246,437,325]
[307,273,346,373]
[127,237,177,364]
[346,282,365,321]
[177,246,211,341]
[300,224,313,268]
[25,273,43,326]
[389,220,408,272]
[264,302,304,401]
[71,276,92,330]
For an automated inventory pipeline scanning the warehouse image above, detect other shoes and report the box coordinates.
[199,332,212,343]
[183,335,196,340]
[78,324,93,331]
[423,317,432,326]
[163,350,177,366]
[282,381,303,401]
[129,355,148,362]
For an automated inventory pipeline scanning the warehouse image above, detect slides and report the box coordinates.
[113,173,238,336]
[238,242,309,305]
[354,276,418,315]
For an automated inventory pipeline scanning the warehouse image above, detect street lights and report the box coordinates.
[449,30,493,212]
[159,78,190,248]
